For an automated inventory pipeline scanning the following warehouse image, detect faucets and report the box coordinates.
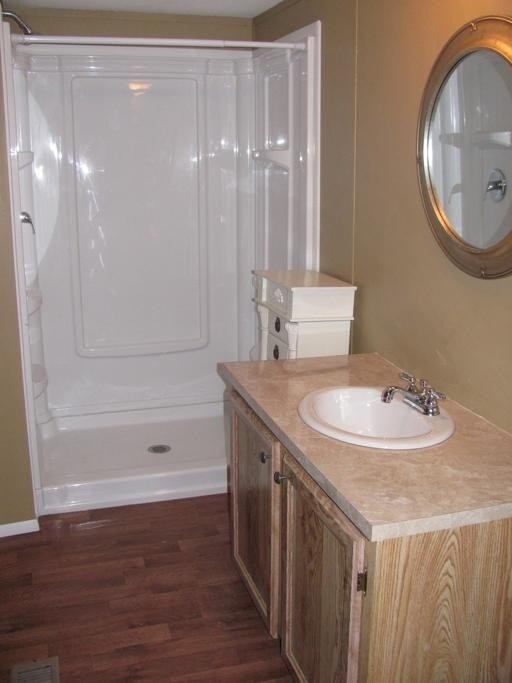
[381,372,446,416]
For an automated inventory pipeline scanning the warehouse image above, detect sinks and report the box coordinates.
[298,385,455,474]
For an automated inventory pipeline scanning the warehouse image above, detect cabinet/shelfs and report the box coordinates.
[251,267,358,362]
[222,385,512,681]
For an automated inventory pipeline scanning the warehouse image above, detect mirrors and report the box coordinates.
[414,13,511,279]
